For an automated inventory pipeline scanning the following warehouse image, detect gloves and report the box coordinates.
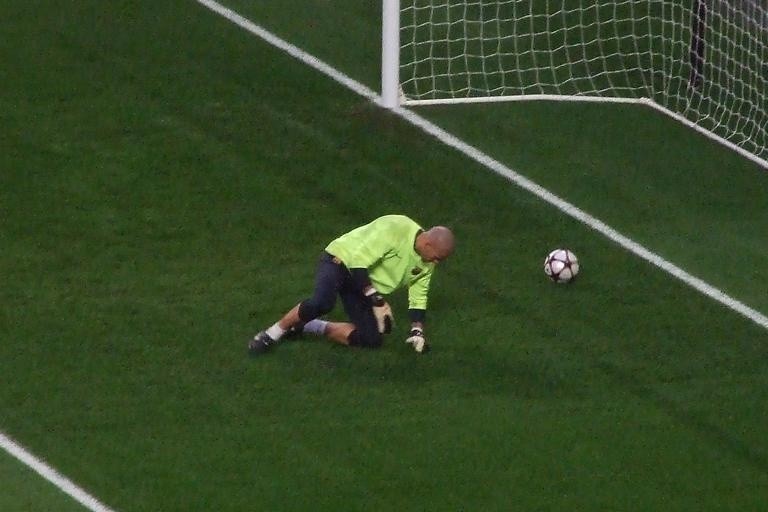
[404,327,425,353]
[364,288,394,333]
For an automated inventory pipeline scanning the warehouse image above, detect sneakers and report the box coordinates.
[248,320,304,353]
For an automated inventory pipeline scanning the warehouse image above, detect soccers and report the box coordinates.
[544,249,580,283]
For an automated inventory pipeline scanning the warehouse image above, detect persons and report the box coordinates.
[248,215,455,354]
